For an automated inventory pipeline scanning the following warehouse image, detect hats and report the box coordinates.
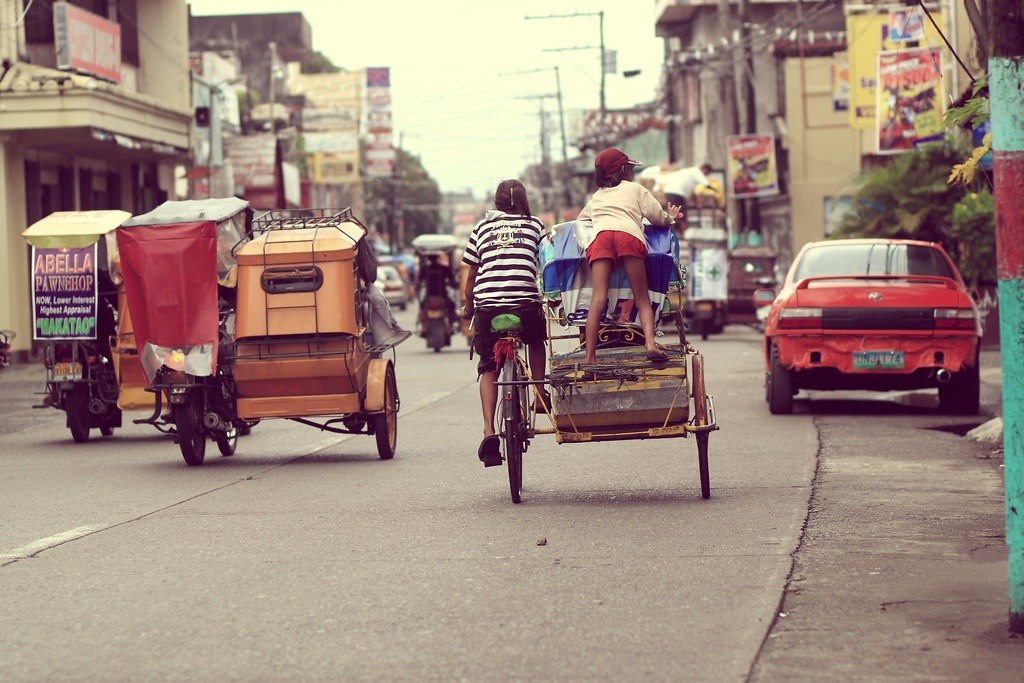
[593,148,639,178]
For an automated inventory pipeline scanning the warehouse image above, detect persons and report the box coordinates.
[575,148,682,376]
[890,12,910,39]
[415,255,459,340]
[162,410,175,422]
[42,268,118,403]
[665,164,718,230]
[461,179,551,462]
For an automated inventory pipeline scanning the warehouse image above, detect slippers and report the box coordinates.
[647,351,670,359]
[530,389,551,414]
[478,435,500,462]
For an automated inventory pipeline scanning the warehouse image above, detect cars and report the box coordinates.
[748,235,985,418]
[372,263,411,312]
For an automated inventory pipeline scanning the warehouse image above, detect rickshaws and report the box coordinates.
[20,206,177,445]
[408,232,476,354]
[457,217,722,507]
[116,195,414,468]
[682,225,775,342]
